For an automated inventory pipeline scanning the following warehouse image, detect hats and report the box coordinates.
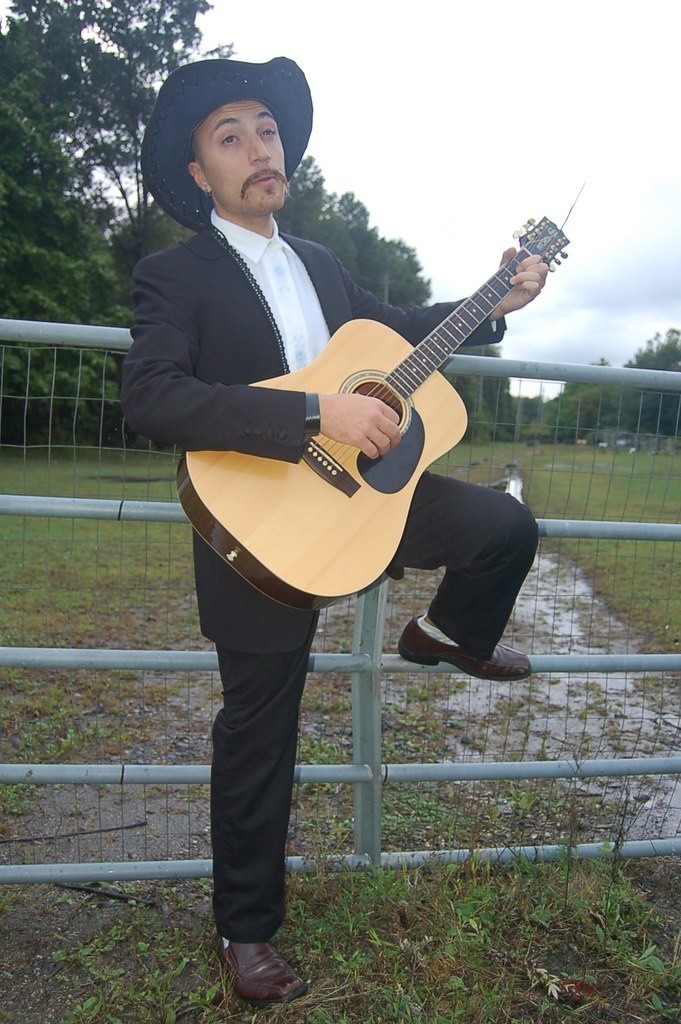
[140,56,314,234]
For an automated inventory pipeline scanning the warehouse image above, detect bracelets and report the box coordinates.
[304,393,321,436]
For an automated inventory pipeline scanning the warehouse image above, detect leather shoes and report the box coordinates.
[398,615,532,681]
[213,934,308,1005]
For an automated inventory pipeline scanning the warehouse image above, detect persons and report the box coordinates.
[119,57,548,1004]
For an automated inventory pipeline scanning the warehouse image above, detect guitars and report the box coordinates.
[175,215,572,608]
